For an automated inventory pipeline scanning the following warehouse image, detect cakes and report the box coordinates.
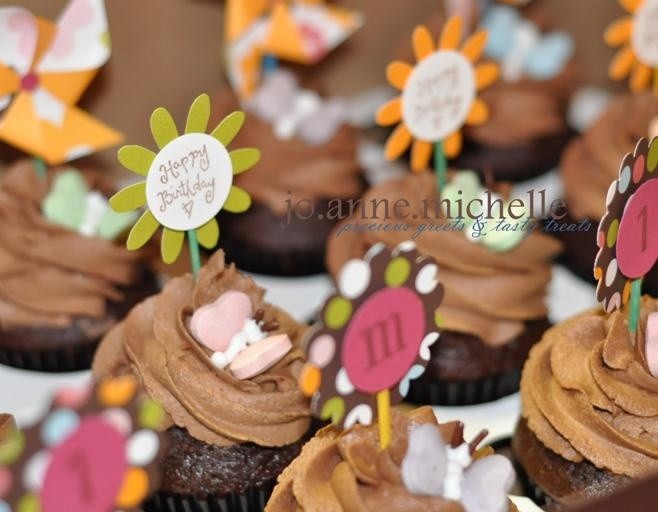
[510,293,658,512]
[212,111,366,278]
[263,409,540,512]
[322,173,552,406]
[463,88,567,181]
[556,93,658,292]
[85,253,321,496]
[0,156,157,373]
